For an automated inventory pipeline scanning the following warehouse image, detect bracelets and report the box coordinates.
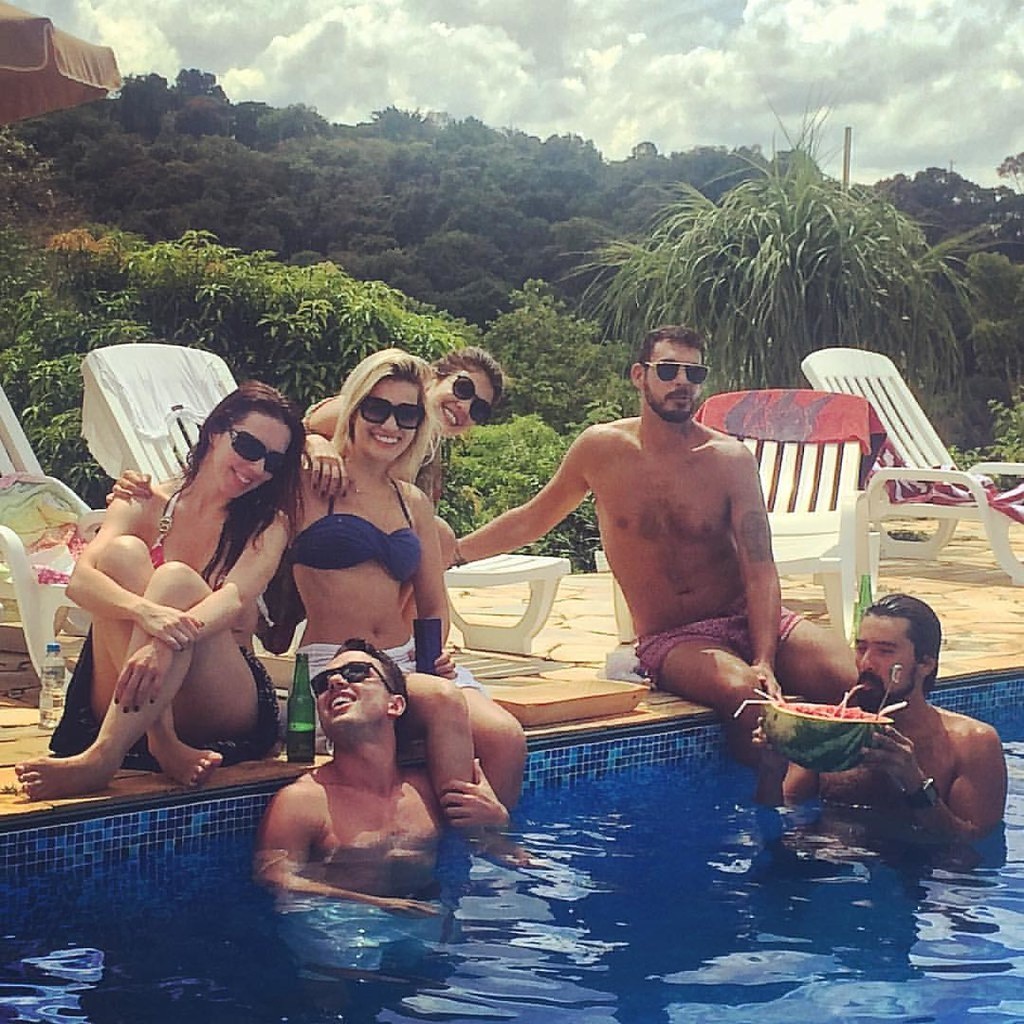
[451,541,467,567]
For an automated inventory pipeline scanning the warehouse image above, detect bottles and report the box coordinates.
[287,653,316,763]
[39,644,66,729]
[854,575,872,642]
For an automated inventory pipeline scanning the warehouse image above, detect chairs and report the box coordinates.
[77,343,578,697]
[0,385,108,704]
[800,347,1024,589]
[594,387,890,650]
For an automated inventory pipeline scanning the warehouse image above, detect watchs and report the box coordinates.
[903,778,940,809]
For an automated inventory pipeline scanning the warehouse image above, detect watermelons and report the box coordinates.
[763,702,895,772]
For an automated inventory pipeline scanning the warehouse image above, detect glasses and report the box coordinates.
[310,661,397,696]
[644,361,709,385]
[359,395,425,430]
[453,374,492,426]
[221,418,288,476]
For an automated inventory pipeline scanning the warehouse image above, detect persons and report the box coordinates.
[783,594,1007,830]
[12,346,531,859]
[452,322,863,770]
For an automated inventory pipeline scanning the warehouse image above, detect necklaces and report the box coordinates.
[348,480,391,495]
